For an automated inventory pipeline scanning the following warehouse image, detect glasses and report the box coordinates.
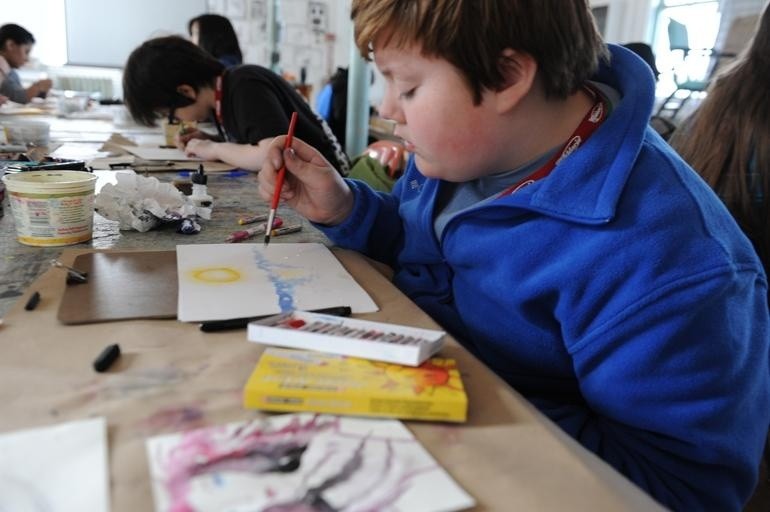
[168,109,181,125]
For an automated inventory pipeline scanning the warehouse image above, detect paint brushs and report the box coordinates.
[264,111,298,246]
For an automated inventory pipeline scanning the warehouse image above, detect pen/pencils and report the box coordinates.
[109,163,176,167]
[271,224,303,237]
[199,306,352,332]
[238,214,268,225]
[270,317,427,347]
[224,218,282,243]
[178,171,249,177]
[51,259,89,282]
[181,122,187,147]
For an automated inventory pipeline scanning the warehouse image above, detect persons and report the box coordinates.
[318,62,379,152]
[121,29,351,179]
[188,14,242,69]
[667,4,769,281]
[621,43,662,83]
[259,1,769,511]
[0,24,52,104]
